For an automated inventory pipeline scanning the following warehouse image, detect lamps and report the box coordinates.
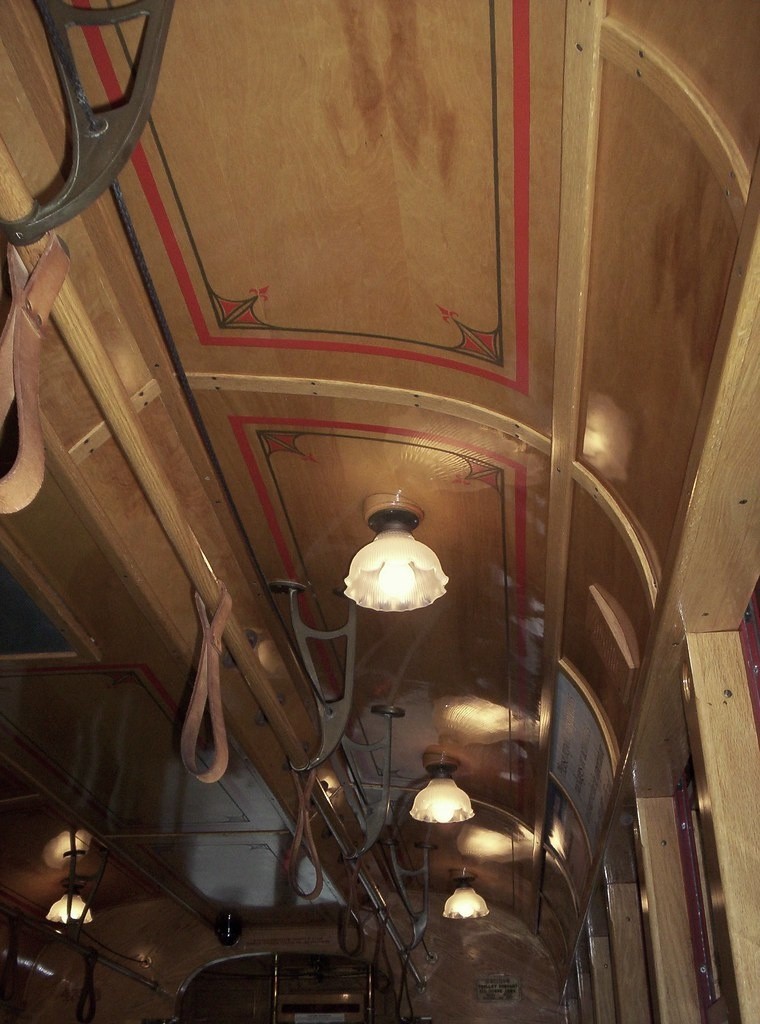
[342,493,449,613]
[44,877,92,926]
[441,874,490,920]
[408,751,475,824]
